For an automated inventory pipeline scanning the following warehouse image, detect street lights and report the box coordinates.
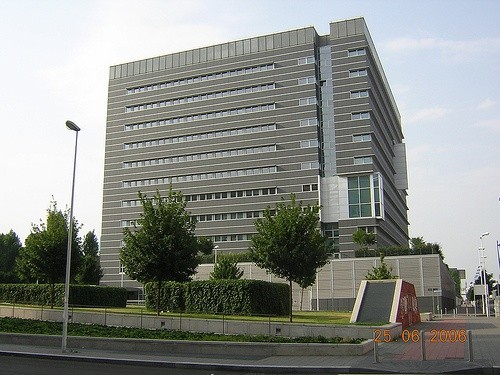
[59,121,81,351]
[475,232,490,316]
